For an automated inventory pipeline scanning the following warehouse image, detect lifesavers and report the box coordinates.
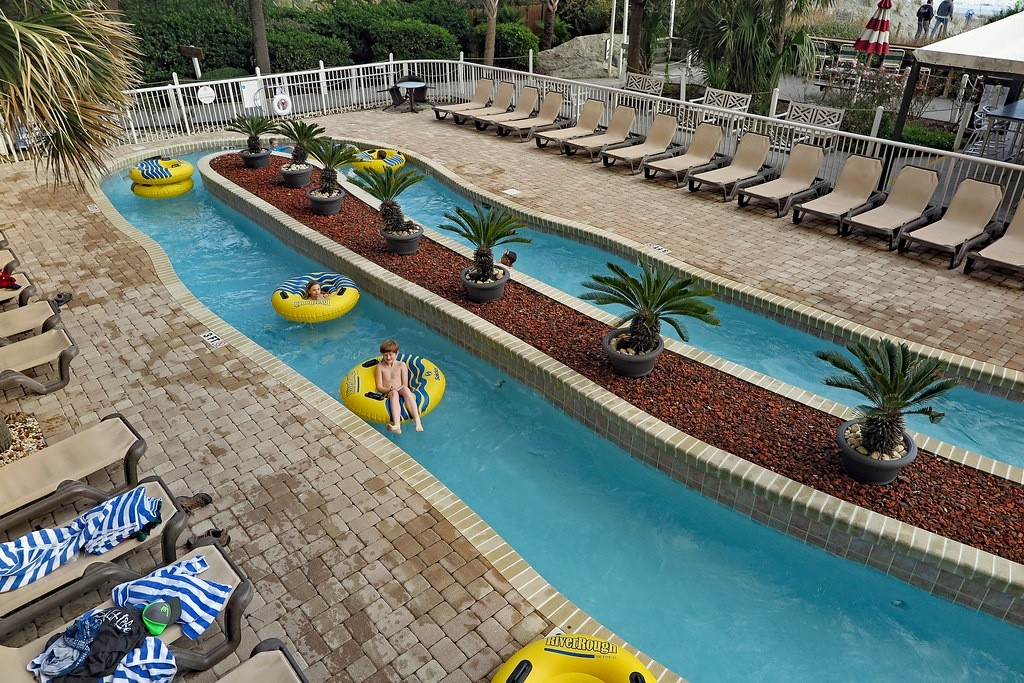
[270,272,362,323]
[340,351,446,425]
[129,158,193,200]
[272,94,293,117]
[306,144,406,171]
[491,634,657,683]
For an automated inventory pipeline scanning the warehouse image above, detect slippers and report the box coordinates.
[51,299,61,313]
[55,292,72,304]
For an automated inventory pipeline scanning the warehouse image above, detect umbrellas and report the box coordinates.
[853,0,893,66]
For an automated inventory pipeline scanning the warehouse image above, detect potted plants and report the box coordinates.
[346,167,424,253]
[812,337,963,485]
[300,140,361,215]
[574,259,720,378]
[441,205,531,300]
[274,119,326,187]
[223,113,280,168]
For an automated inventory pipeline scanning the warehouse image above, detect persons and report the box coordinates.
[306,280,330,300]
[375,340,424,434]
[269,138,294,152]
[377,150,386,160]
[917,0,933,39]
[931,0,954,37]
[501,250,517,266]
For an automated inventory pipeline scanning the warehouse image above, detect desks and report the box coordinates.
[811,83,876,107]
[986,99,1024,165]
[398,81,426,114]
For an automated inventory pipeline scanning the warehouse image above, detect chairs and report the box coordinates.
[432,78,1024,281]
[1,221,309,683]
[952,105,1007,168]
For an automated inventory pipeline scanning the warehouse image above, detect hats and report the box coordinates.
[141,597,180,635]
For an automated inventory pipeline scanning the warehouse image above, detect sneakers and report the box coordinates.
[186,528,230,550]
[176,493,213,514]
